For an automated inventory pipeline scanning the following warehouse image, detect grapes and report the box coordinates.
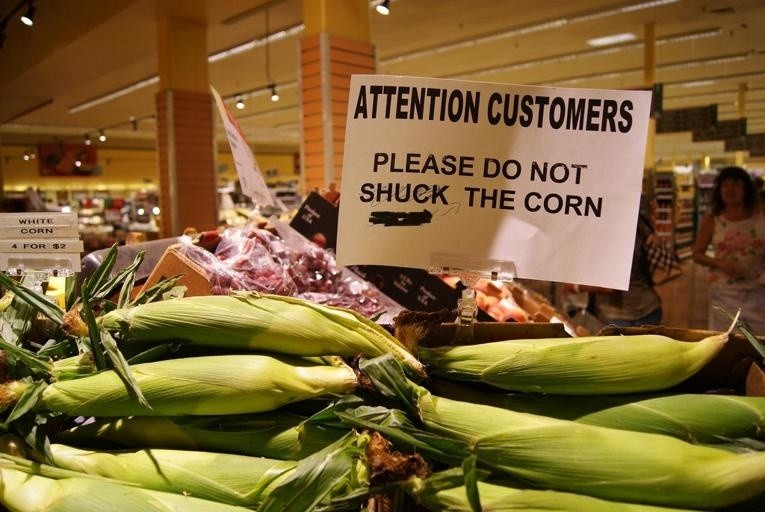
[208,249,384,316]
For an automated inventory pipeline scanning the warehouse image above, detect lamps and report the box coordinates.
[375,0,392,15]
[20,1,36,26]
[268,84,280,102]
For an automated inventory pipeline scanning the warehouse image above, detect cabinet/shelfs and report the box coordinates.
[641,171,718,262]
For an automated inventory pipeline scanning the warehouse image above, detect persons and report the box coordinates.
[691,166,764,337]
[564,217,664,327]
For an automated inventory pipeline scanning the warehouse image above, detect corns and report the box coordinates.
[0,292,765,512]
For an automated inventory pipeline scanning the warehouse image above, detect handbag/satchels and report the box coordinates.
[639,216,682,287]
